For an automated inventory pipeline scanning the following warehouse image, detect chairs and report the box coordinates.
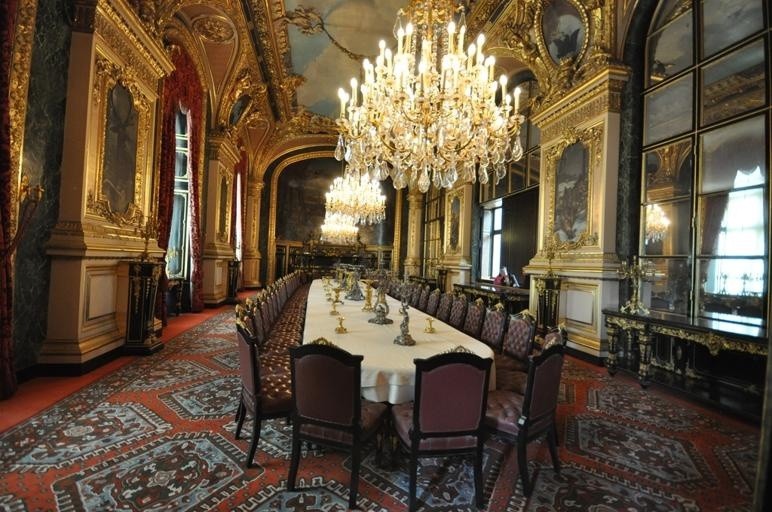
[245,270,311,355]
[436,291,453,323]
[418,285,430,312]
[285,338,387,509]
[391,346,495,512]
[235,321,295,469]
[461,298,487,340]
[426,288,441,316]
[480,301,509,347]
[497,312,537,368]
[233,304,292,425]
[501,323,568,447]
[486,344,569,499]
[447,294,468,329]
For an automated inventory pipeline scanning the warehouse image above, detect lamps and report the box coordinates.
[335,2,523,193]
[321,215,360,246]
[326,172,388,225]
[644,204,671,245]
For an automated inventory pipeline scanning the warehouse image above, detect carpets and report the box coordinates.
[0,294,762,512]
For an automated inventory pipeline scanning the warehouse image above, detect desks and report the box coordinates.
[453,281,529,313]
[604,306,767,429]
